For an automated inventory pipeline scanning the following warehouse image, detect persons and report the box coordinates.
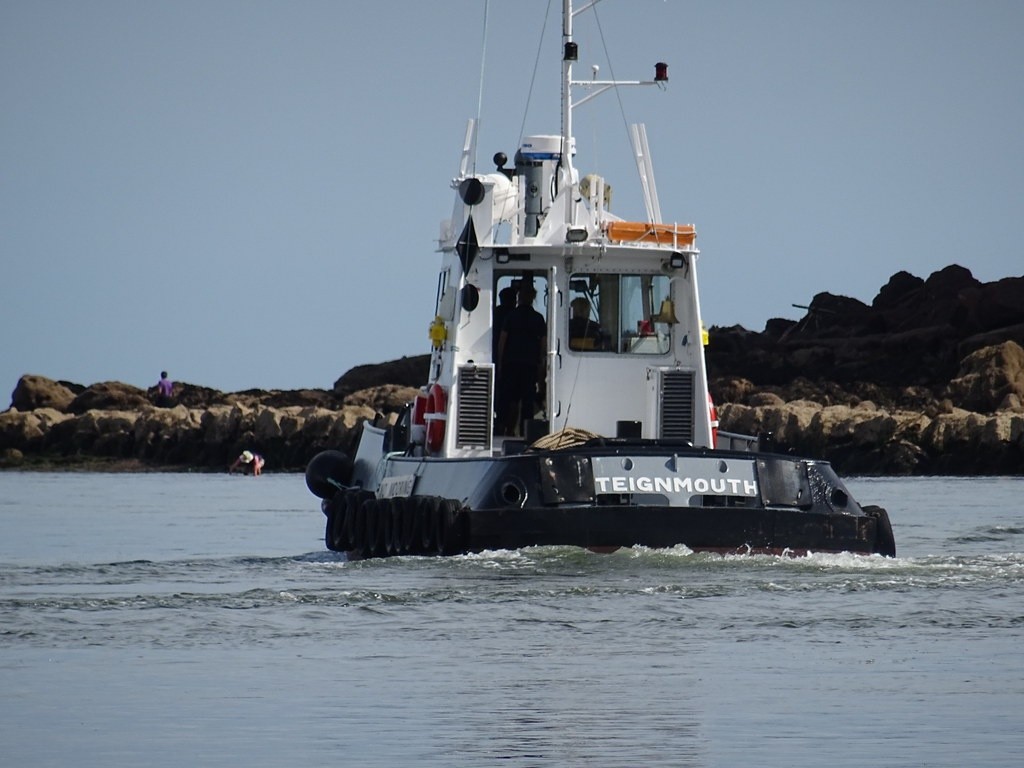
[229,451,265,477]
[158,372,172,407]
[494,287,548,437]
[569,297,604,352]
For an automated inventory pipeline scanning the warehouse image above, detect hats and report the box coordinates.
[240,451,254,462]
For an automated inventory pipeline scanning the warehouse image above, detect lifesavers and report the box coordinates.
[423,382,446,453]
[306,448,355,499]
[324,489,463,561]
[871,507,897,558]
[861,505,881,515]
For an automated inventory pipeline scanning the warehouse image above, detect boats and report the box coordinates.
[300,1,901,560]
